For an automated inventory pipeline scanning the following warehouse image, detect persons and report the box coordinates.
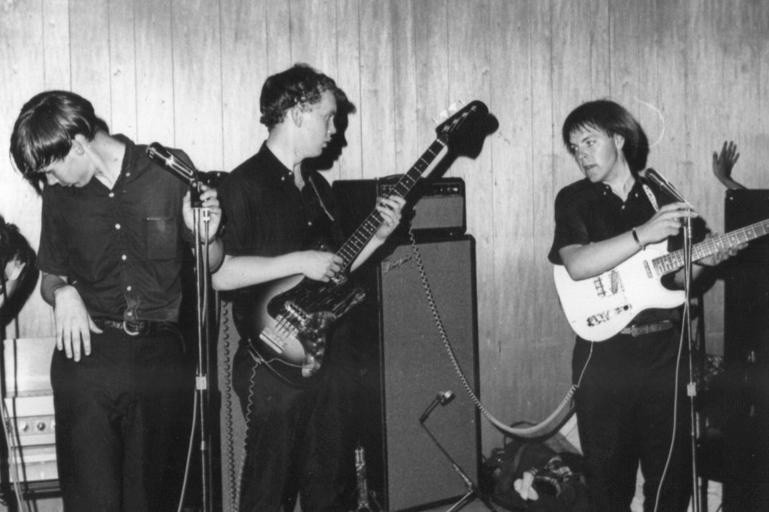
[712,140,743,191]
[12,91,225,512]
[210,65,408,512]
[549,104,748,511]
[2,221,40,511]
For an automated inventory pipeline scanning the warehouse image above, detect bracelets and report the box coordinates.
[631,226,645,250]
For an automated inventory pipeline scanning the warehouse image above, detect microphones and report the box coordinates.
[144,141,208,191]
[645,167,691,214]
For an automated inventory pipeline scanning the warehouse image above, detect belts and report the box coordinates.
[623,321,677,336]
[93,315,183,338]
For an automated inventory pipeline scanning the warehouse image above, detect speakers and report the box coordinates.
[723,189,769,512]
[337,237,482,512]
[207,293,250,512]
[333,177,466,241]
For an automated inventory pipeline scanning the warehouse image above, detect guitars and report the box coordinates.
[554,221,769,342]
[231,99,490,390]
